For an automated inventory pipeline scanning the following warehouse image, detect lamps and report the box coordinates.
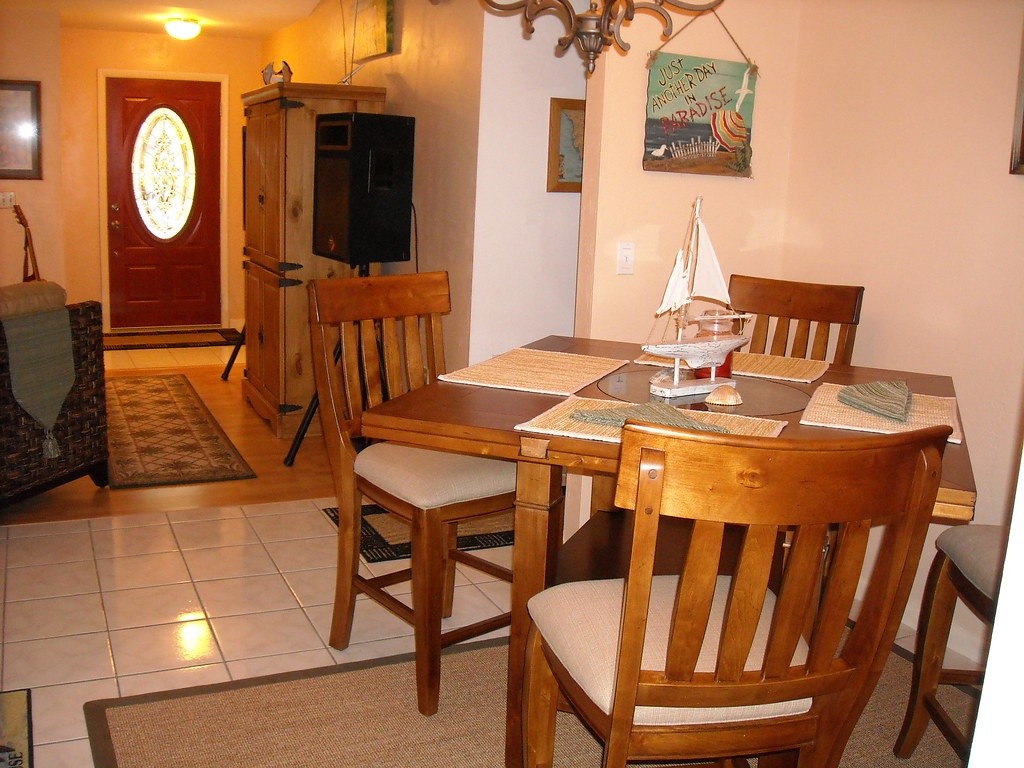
[165,15,203,40]
[480,0,723,75]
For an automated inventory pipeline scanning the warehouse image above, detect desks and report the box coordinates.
[360,334,978,767]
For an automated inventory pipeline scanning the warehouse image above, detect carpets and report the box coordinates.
[106,373,258,490]
[323,504,514,563]
[0,686,34,768]
[83,619,982,767]
[102,328,247,350]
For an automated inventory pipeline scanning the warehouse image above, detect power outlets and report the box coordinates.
[0,192,16,208]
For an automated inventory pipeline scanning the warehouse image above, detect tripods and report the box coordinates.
[284,264,394,470]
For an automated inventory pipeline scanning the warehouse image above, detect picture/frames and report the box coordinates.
[1003,40,1024,175]
[545,97,584,195]
[0,78,44,181]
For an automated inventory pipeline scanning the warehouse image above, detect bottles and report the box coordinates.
[692,309,734,379]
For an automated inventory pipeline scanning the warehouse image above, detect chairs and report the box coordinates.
[892,522,1013,767]
[726,275,865,364]
[305,271,519,715]
[519,420,958,768]
[0,266,108,509]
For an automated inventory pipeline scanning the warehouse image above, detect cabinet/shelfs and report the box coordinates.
[240,80,388,439]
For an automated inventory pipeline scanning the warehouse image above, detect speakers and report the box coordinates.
[310,111,416,268]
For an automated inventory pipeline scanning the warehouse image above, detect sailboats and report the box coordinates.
[630,193,753,368]
